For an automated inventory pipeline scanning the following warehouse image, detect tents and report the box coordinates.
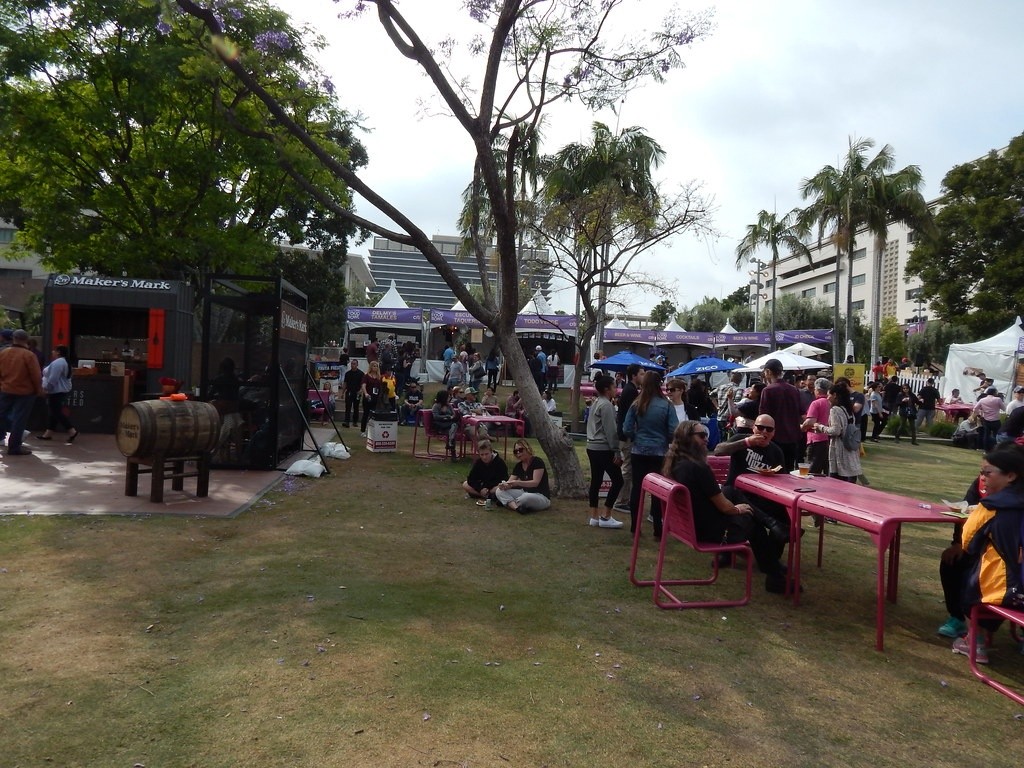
[656,317,714,355]
[776,327,833,355]
[942,315,1024,414]
[713,318,771,354]
[343,280,424,372]
[424,288,503,373]
[516,287,583,346]
[589,316,656,373]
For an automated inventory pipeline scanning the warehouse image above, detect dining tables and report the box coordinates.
[734,474,967,650]
[461,415,524,461]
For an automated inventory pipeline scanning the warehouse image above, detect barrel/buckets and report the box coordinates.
[116,398,221,457]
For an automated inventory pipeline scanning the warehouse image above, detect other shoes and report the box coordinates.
[65,431,80,446]
[353,423,359,427]
[342,423,350,428]
[451,451,458,462]
[495,500,503,507]
[446,446,453,456]
[37,435,52,441]
[9,448,31,454]
[516,506,528,514]
[765,575,804,594]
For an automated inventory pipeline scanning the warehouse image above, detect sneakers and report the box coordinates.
[614,504,632,513]
[598,516,624,528]
[953,624,991,665]
[940,616,969,636]
[590,518,599,527]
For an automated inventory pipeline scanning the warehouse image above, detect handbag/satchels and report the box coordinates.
[906,404,917,419]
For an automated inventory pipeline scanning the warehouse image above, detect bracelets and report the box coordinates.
[734,506,741,514]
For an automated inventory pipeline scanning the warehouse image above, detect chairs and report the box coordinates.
[307,383,1024,705]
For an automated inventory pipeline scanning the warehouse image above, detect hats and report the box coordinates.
[760,359,783,373]
[983,440,1024,475]
[465,387,479,395]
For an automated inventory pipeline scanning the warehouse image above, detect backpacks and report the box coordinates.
[838,406,861,451]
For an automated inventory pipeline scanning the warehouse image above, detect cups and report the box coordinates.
[905,397,909,401]
[815,422,822,434]
[482,408,486,416]
[472,413,476,418]
[798,463,811,475]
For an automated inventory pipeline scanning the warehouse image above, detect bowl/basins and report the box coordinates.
[463,416,467,418]
[937,398,946,404]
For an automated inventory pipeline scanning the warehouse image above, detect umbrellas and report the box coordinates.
[662,352,746,378]
[588,349,667,370]
[731,349,831,373]
[845,339,856,363]
[784,341,829,356]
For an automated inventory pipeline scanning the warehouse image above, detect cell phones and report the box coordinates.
[795,487,816,493]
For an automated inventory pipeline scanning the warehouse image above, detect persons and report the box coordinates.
[122,340,134,357]
[844,354,943,446]
[585,359,865,595]
[495,438,551,512]
[321,335,558,461]
[463,440,509,501]
[932,371,1024,663]
[0,330,79,455]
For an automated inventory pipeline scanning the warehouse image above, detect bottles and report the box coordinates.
[485,499,491,510]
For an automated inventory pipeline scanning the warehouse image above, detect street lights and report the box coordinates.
[911,284,927,323]
[749,256,769,331]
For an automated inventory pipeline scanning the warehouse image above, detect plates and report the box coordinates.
[465,415,472,417]
[476,501,486,505]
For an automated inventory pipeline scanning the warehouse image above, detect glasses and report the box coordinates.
[755,424,775,433]
[694,432,709,437]
[512,447,525,453]
[458,391,464,394]
[664,388,679,393]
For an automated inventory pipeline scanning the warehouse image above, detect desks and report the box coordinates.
[933,404,974,424]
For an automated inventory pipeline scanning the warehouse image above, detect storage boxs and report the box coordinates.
[366,419,398,452]
